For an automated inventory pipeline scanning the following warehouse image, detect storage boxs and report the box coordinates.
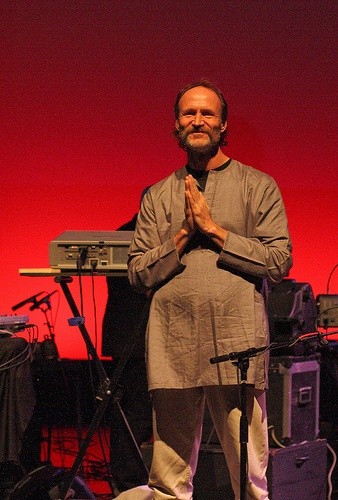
[140,438,327,500]
[202,354,321,447]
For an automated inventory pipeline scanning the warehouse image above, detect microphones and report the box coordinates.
[30,294,50,311]
[13,324,34,330]
[12,293,40,310]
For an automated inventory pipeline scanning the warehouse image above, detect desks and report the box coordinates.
[18,267,151,500]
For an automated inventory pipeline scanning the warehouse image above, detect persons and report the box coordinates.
[100,184,154,492]
[126,80,294,500]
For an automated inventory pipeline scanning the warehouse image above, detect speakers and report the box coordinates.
[7,464,96,500]
